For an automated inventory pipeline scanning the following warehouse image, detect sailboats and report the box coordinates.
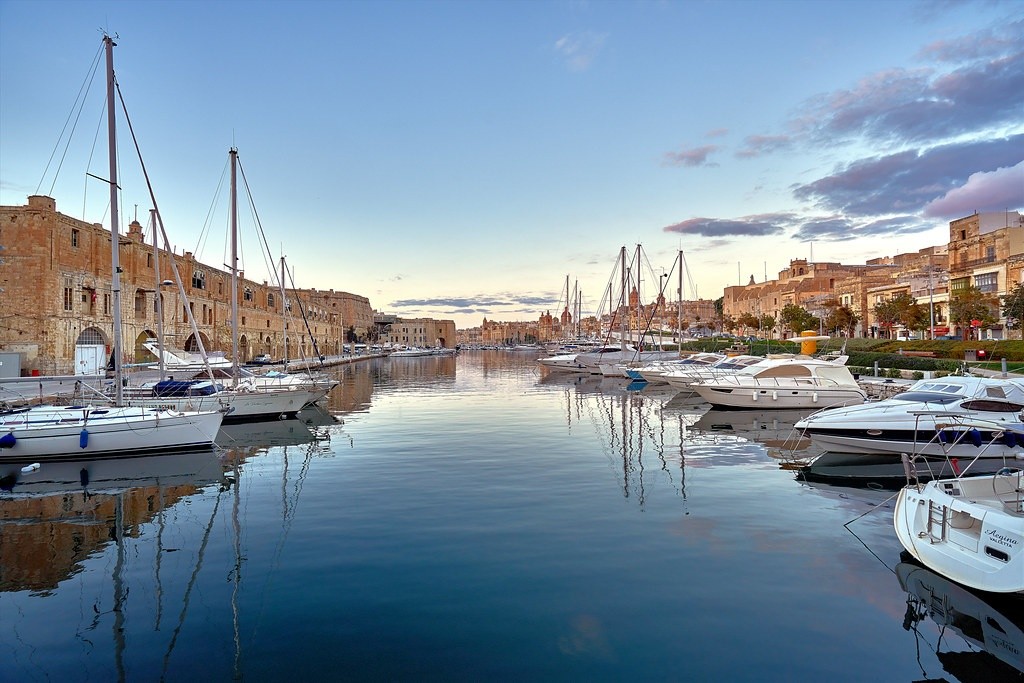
[537,238,749,381]
[0,12,341,460]
[537,370,713,514]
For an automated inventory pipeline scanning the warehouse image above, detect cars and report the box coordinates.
[693,334,708,339]
[935,336,962,340]
[908,336,919,341]
[721,334,758,341]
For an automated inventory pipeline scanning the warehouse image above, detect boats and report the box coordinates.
[626,353,869,409]
[686,423,1024,507]
[388,347,457,356]
[843,525,1024,675]
[793,280,1024,593]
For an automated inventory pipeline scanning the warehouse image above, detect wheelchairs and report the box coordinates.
[1,404,343,683]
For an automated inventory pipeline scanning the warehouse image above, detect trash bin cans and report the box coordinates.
[978,350,987,361]
[32,370,39,376]
[964,349,976,361]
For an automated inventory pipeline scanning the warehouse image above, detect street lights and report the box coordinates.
[155,279,173,381]
[660,274,667,346]
